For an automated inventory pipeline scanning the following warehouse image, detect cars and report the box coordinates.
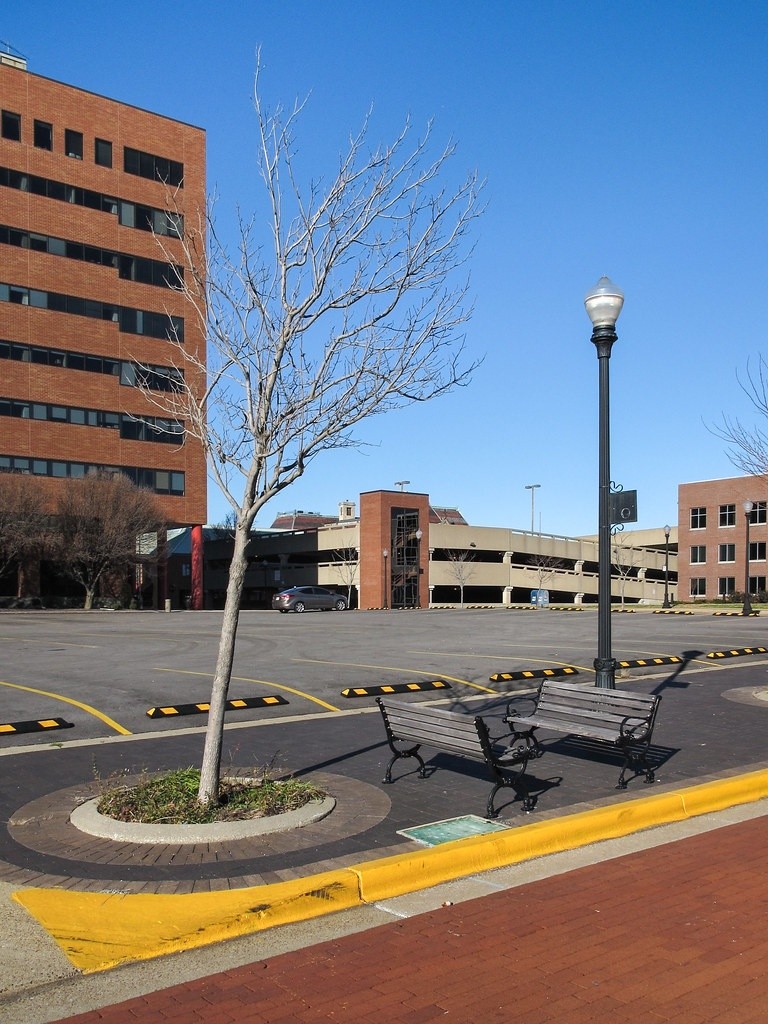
[272,586,348,613]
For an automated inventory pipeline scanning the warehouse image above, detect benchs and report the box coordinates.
[376,696,547,819]
[501,678,663,789]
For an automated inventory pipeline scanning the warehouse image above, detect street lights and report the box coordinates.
[415,529,422,608]
[525,485,540,536]
[585,276,624,689]
[383,548,388,608]
[663,524,670,608]
[395,480,409,492]
[743,500,753,612]
